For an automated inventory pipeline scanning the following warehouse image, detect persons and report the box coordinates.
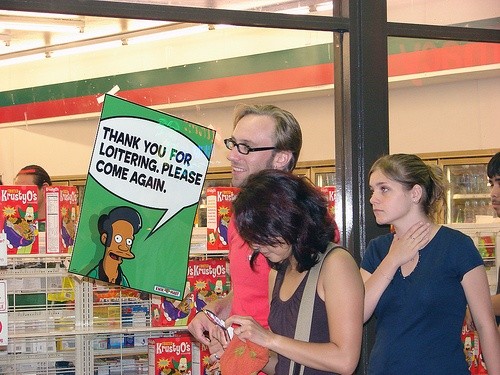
[186,104,342,375]
[209,170,365,375]
[465,150,500,374]
[13,165,54,231]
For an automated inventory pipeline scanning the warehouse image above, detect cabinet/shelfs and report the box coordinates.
[0,149,500,375]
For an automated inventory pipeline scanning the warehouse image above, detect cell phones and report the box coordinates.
[203,309,225,329]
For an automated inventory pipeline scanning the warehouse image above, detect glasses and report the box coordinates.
[224,138,278,155]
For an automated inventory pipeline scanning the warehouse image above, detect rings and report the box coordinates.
[356,153,500,375]
[214,352,220,360]
[410,234,416,240]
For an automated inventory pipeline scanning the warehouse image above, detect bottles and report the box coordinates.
[456,173,487,193]
[455,201,497,223]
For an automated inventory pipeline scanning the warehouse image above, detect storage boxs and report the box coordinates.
[0,184,488,375]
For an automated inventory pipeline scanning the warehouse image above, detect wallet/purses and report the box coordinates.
[219,334,269,375]
